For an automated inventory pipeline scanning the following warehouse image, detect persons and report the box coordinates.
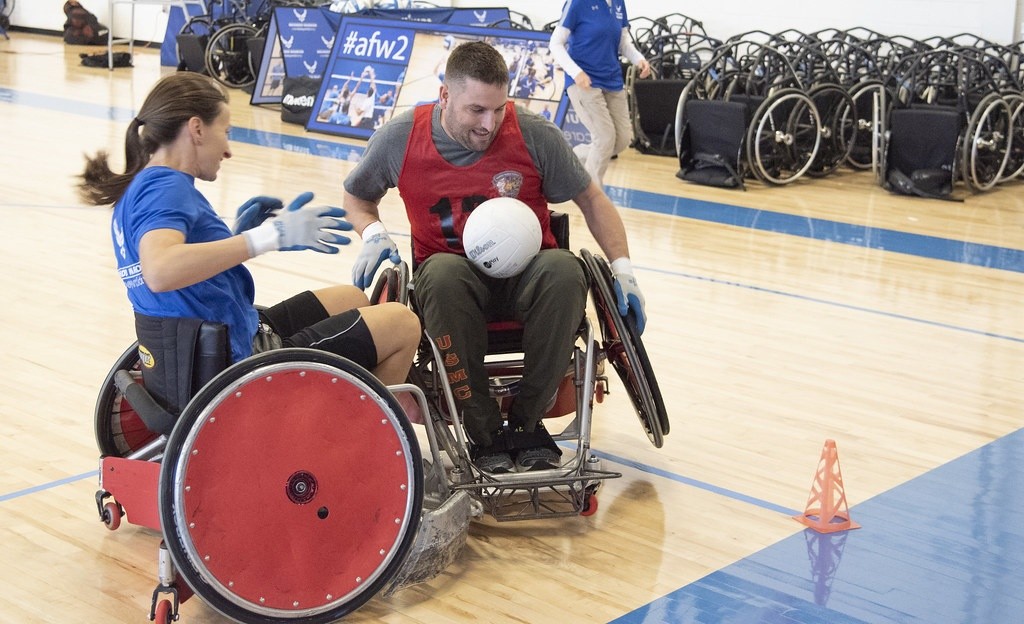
[320,69,404,129]
[549,0,650,189]
[77,73,422,399]
[343,42,647,474]
[433,36,455,83]
[492,38,555,120]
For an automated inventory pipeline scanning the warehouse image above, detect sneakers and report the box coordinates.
[461,410,518,473]
[507,401,563,472]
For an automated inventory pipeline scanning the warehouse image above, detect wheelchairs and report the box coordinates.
[96,326,485,624]
[370,207,671,523]
[595,12,1024,203]
[175,0,340,91]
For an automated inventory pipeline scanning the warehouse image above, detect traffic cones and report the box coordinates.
[793,439,861,534]
[803,525,849,606]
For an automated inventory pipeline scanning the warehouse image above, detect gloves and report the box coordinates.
[610,257,647,337]
[242,192,354,258]
[231,195,284,236]
[351,220,402,292]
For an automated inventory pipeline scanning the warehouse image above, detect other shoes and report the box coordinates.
[422,475,483,517]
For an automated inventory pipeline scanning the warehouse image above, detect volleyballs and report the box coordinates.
[462,196,543,280]
